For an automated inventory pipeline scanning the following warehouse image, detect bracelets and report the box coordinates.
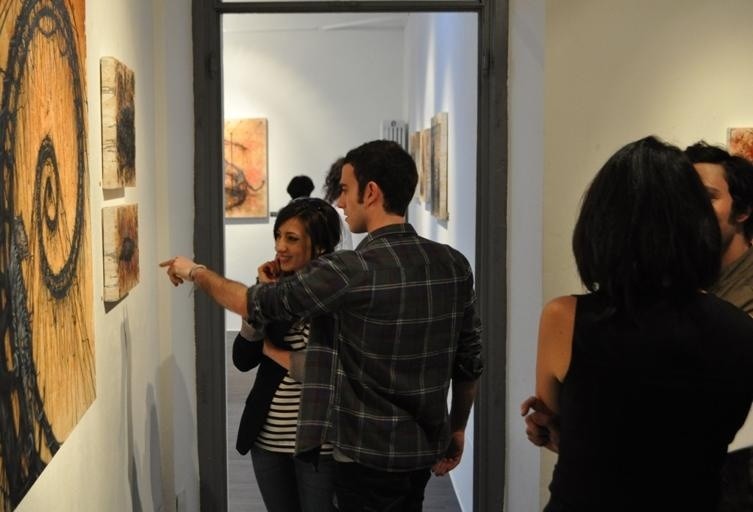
[189,264,206,281]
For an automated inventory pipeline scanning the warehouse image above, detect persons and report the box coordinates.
[519,136,753,512]
[232,201,342,512]
[160,138,485,512]
[285,175,317,197]
[324,156,368,251]
[684,142,753,326]
